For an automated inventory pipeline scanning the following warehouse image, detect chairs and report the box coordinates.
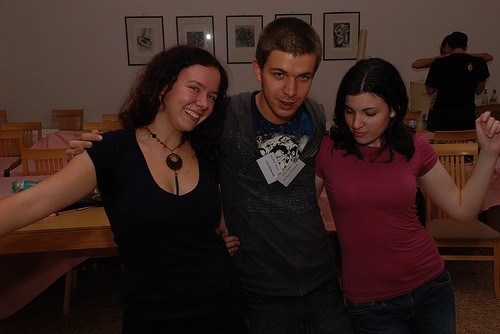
[51,108,84,131]
[20,148,66,176]
[434,130,481,163]
[0,121,43,157]
[0,131,24,157]
[425,142,500,300]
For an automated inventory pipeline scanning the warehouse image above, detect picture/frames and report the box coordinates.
[124,15,165,66]
[176,15,215,57]
[226,15,264,64]
[323,11,361,61]
[275,14,313,26]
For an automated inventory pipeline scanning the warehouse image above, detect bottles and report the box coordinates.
[481,89,497,105]
[12,181,38,192]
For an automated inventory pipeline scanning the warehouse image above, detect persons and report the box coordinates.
[65,17,353,334]
[223,57,500,334]
[411,31,493,161]
[0,46,229,334]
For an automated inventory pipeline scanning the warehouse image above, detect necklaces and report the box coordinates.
[145,126,186,171]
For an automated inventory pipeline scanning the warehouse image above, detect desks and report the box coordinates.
[0,176,336,320]
[0,156,71,177]
[416,156,500,222]
